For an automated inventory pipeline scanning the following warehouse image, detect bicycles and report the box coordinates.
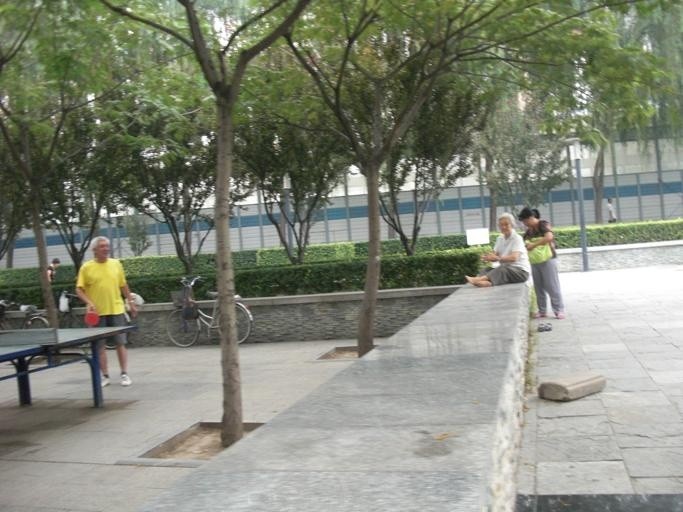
[166,275,254,348]
[0,290,130,350]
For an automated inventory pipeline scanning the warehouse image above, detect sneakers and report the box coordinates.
[532,311,547,319]
[537,323,552,332]
[556,312,565,319]
[120,375,131,386]
[101,377,110,387]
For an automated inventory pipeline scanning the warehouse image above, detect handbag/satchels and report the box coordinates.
[525,237,553,266]
[183,304,199,321]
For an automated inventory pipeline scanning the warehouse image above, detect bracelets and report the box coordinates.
[128,300,134,304]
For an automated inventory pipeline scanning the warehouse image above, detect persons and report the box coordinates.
[518,208,565,320]
[75,235,137,386]
[465,213,529,288]
[607,198,616,224]
[47,257,60,283]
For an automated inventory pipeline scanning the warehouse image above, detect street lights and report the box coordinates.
[567,136,589,273]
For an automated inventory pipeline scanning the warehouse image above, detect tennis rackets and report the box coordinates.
[84,307,98,325]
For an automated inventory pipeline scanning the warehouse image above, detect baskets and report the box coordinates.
[170,290,185,308]
